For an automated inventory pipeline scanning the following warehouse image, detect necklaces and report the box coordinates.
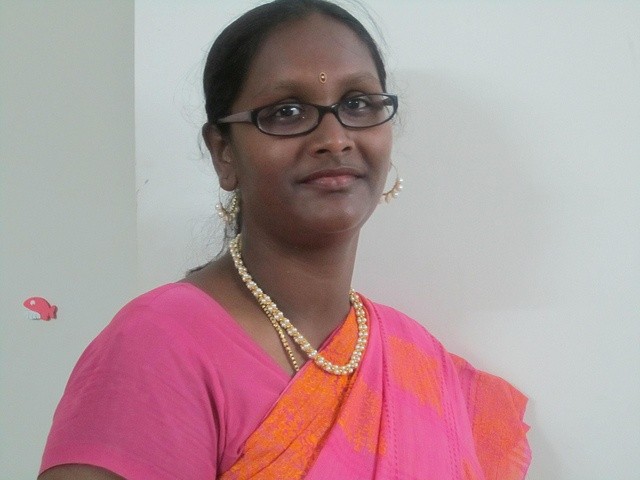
[230,231,369,376]
[234,231,302,373]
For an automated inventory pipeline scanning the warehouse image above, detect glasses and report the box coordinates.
[215,91,398,138]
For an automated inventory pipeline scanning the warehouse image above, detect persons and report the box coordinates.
[37,0,532,480]
